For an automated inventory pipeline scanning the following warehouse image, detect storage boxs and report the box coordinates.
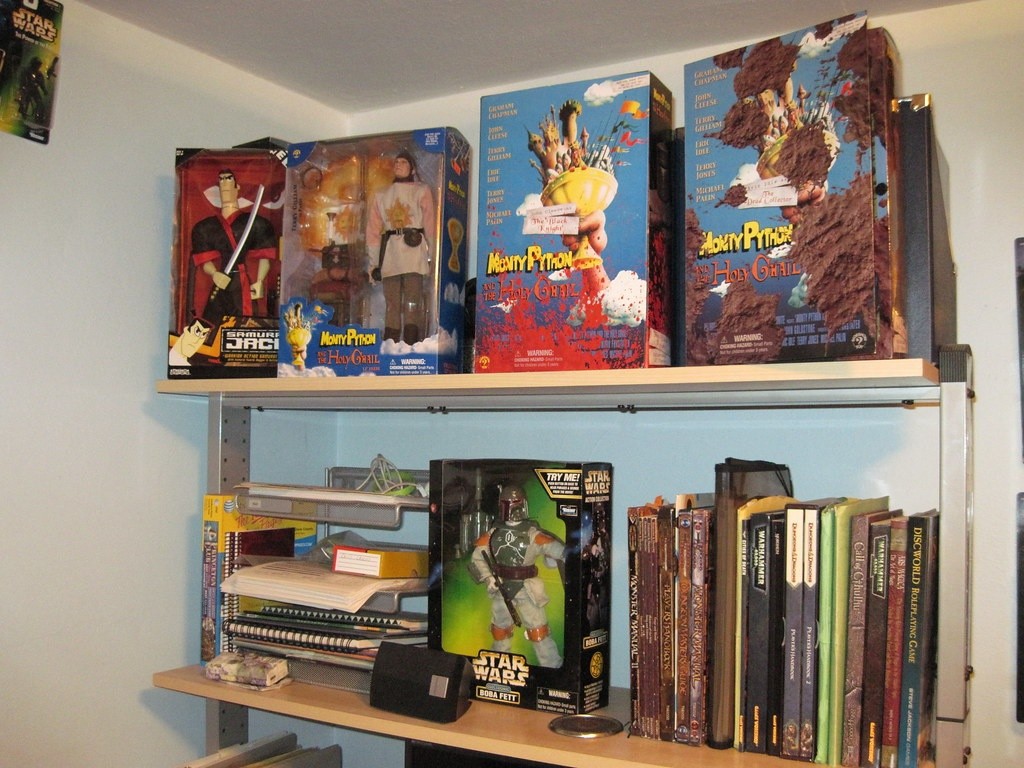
[428,458,614,713]
[865,26,908,359]
[278,126,472,376]
[472,70,675,374]
[167,147,287,380]
[331,544,428,578]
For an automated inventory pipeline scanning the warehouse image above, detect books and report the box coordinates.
[626,494,973,768]
[202,495,428,692]
[238,744,343,768]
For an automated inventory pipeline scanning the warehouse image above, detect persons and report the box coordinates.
[365,153,435,346]
[470,486,567,668]
[17,56,47,123]
[191,167,278,322]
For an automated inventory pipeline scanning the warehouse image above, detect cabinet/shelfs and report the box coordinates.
[153,343,977,768]
